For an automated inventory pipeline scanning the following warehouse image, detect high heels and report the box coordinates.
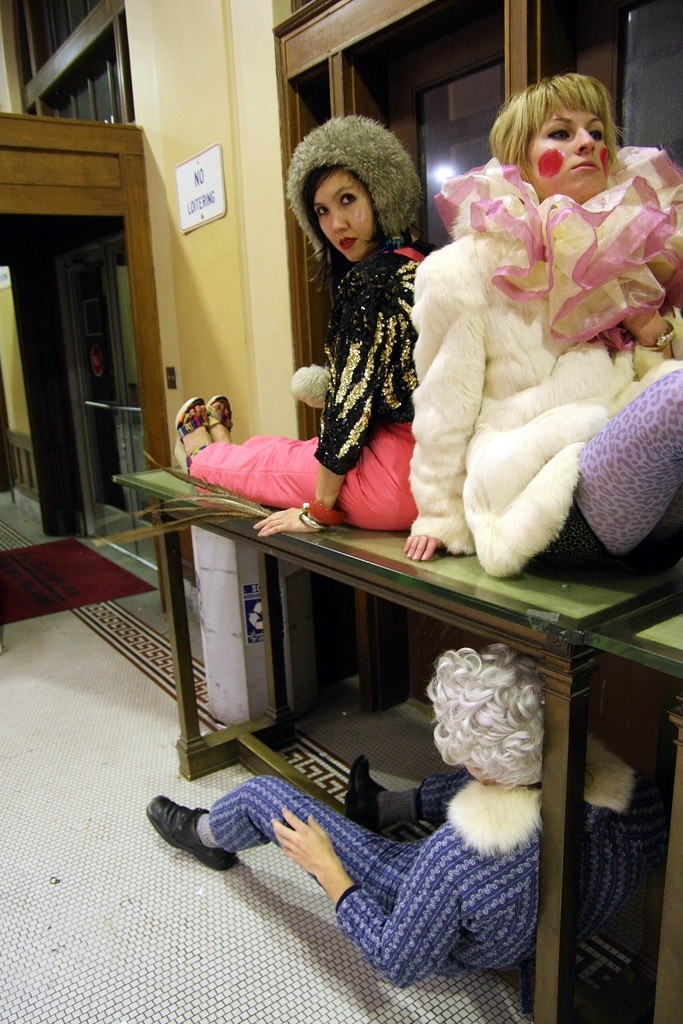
[174,395,231,476]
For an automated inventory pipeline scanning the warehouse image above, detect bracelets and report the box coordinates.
[298,500,348,530]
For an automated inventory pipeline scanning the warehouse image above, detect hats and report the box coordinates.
[286,115,423,265]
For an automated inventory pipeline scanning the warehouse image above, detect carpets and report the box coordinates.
[0,536,158,625]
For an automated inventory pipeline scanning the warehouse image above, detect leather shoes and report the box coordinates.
[344,754,386,826]
[146,795,239,870]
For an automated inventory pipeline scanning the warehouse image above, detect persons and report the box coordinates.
[146,643,646,989]
[176,116,422,537]
[403,73,683,583]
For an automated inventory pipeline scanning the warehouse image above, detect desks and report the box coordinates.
[112,468,683,1024]
